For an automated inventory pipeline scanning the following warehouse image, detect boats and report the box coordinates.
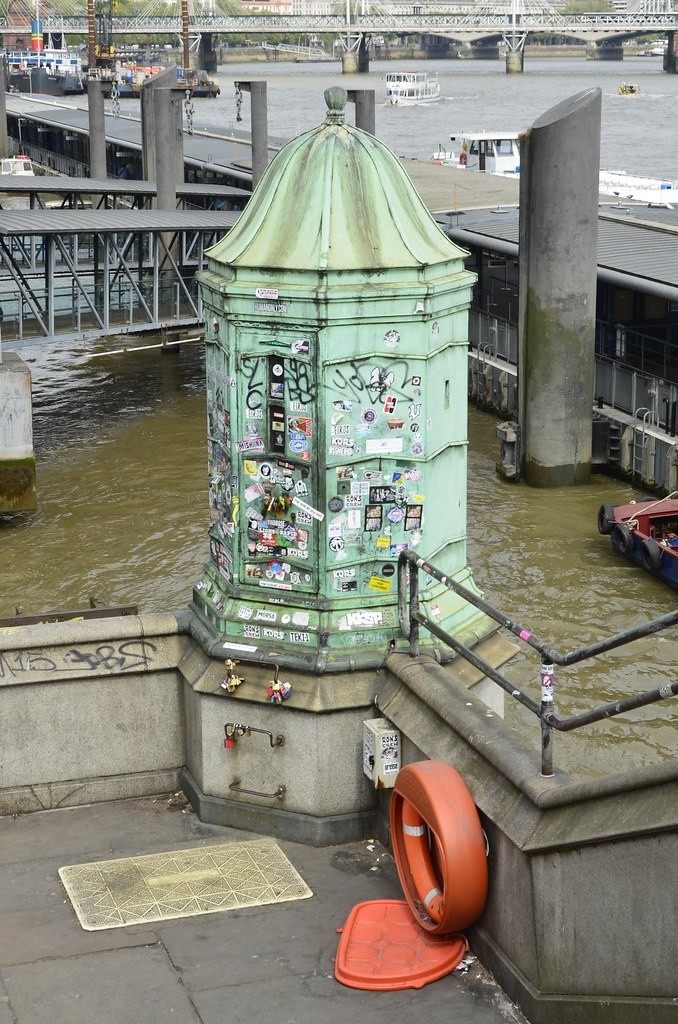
[599,169,678,205]
[381,71,442,105]
[597,494,678,592]
[428,132,521,181]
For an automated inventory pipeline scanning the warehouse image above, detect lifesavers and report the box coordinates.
[401,797,450,925]
